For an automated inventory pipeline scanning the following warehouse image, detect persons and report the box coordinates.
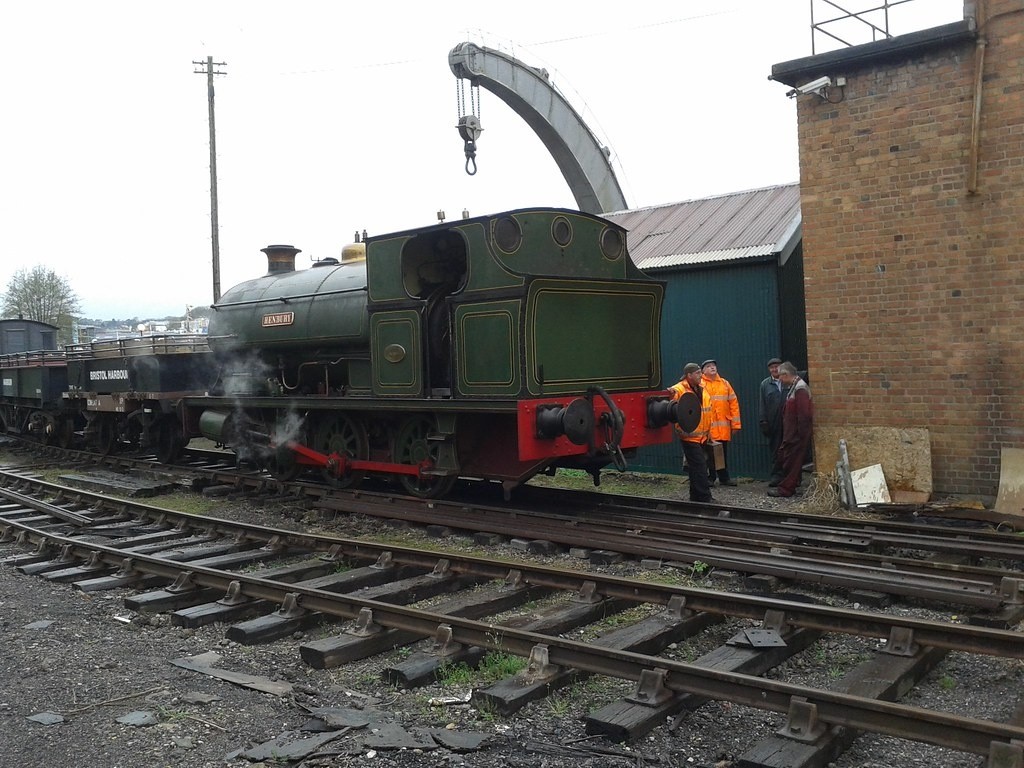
[700,359,742,487]
[668,363,723,504]
[759,358,812,496]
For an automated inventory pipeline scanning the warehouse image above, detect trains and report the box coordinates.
[1,207,700,513]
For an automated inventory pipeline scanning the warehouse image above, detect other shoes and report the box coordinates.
[767,487,796,497]
[690,497,723,505]
[707,479,715,487]
[719,479,737,486]
[768,480,782,486]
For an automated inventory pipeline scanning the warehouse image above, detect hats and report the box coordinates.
[767,358,782,366]
[701,360,716,368]
[680,363,701,380]
